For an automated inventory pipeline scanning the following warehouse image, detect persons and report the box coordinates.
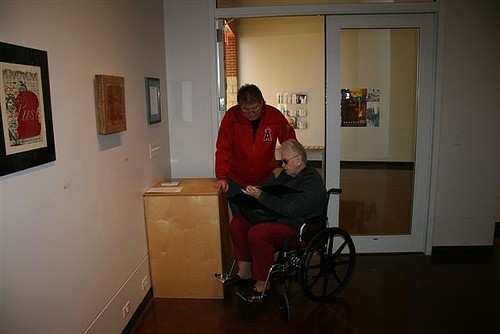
[215,83,295,219]
[224,138,325,301]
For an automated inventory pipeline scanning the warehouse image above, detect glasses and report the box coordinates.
[242,105,262,114]
[280,154,300,164]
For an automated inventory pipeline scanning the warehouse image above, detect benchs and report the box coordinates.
[275,146,326,150]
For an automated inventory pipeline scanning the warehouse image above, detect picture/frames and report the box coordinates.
[145,77,161,124]
[0,41,56,177]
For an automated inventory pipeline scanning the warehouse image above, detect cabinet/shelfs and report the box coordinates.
[143,178,235,299]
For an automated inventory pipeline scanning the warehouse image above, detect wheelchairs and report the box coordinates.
[213,188,357,323]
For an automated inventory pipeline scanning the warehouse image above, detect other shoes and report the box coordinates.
[226,274,251,288]
[241,287,271,297]
